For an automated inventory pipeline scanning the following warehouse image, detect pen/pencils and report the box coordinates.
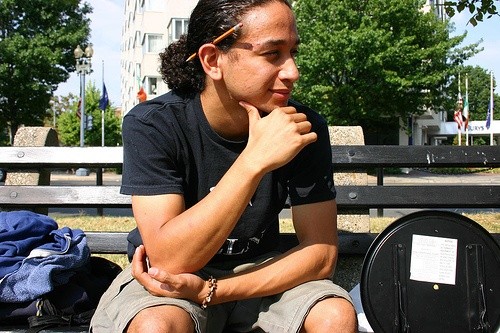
[185,23,243,64]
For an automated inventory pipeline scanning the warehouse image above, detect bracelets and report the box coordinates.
[199,278,217,309]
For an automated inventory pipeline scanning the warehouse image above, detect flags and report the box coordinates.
[461,94,471,130]
[97,83,109,111]
[136,86,147,104]
[486,99,490,128]
[453,91,463,129]
[76,94,82,120]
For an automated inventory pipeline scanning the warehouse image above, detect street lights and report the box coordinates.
[74,43,94,176]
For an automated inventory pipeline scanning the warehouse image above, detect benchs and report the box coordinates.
[0,126,500,333]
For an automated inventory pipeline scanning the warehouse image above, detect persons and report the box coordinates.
[87,0,360,333]
